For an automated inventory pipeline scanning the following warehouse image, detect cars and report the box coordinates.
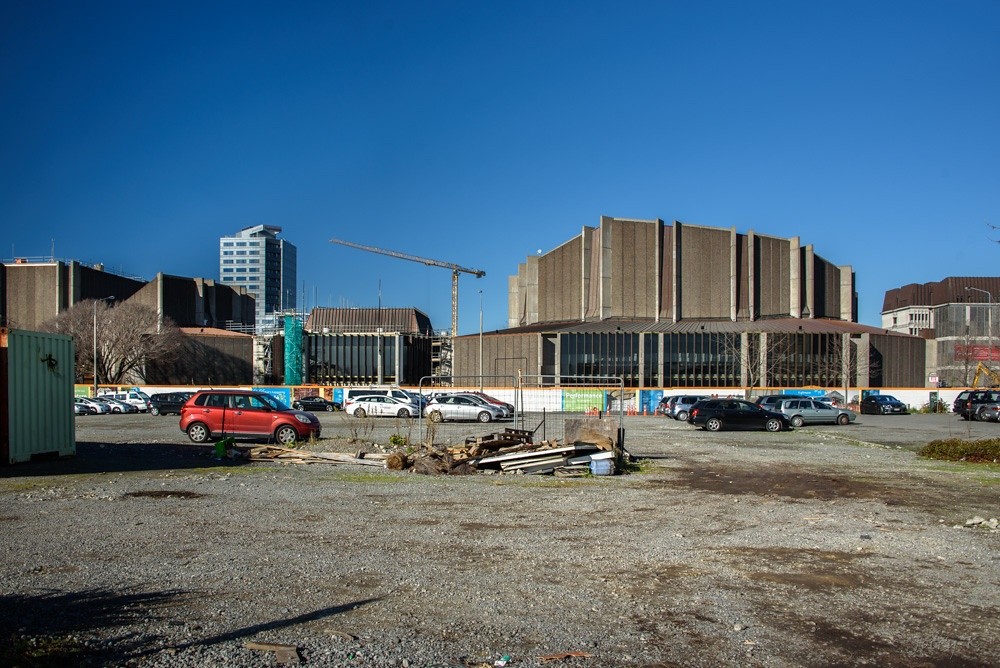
[73,397,138,416]
[179,389,322,447]
[423,395,504,423]
[952,390,1000,423]
[293,396,341,411]
[408,392,514,418]
[808,396,838,408]
[345,395,420,419]
[859,395,907,415]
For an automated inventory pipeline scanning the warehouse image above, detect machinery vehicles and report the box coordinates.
[972,362,1000,388]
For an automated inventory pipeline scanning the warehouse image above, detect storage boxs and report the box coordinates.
[591,460,616,476]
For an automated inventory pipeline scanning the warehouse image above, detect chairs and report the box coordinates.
[239,399,246,408]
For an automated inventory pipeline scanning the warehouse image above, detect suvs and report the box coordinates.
[686,398,791,432]
[147,391,197,416]
[754,395,812,411]
[657,394,713,422]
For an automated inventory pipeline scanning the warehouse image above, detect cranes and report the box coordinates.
[328,238,487,338]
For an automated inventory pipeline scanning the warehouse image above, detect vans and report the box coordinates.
[346,388,418,405]
[775,399,857,428]
[98,390,150,413]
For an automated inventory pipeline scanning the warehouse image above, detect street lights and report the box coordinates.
[94,296,115,397]
[478,290,484,392]
[965,287,992,371]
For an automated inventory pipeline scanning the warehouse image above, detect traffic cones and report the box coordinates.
[643,405,665,417]
[584,405,600,417]
[626,405,638,417]
